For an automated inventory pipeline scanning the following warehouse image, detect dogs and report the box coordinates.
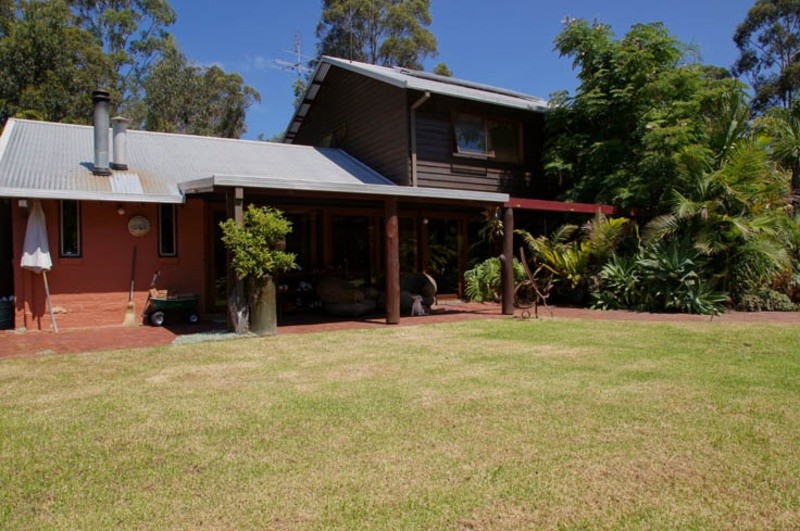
[411,295,424,315]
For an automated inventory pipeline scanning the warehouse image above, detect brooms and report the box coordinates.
[121,244,137,324]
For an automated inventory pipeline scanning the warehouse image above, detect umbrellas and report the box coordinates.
[20,200,57,332]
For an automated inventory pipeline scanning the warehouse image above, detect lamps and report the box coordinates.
[419,215,428,224]
[116,203,125,215]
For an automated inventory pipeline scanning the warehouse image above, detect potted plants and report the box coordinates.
[222,201,302,285]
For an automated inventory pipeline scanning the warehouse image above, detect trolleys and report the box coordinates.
[141,269,203,328]
